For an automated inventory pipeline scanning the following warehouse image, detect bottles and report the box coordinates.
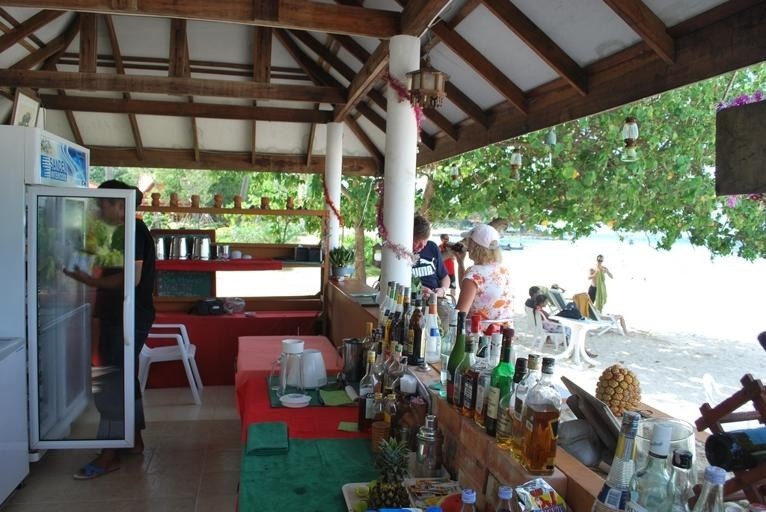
[381,281,397,339]
[392,285,405,346]
[383,394,399,438]
[357,350,382,433]
[520,359,562,476]
[592,412,640,512]
[381,341,398,393]
[403,292,417,356]
[439,355,449,398]
[625,423,675,512]
[460,487,478,512]
[387,344,405,394]
[657,449,695,512]
[385,283,400,342]
[447,312,467,405]
[461,336,491,418]
[510,354,541,462]
[361,322,373,369]
[468,315,480,356]
[496,486,513,512]
[378,281,391,328]
[452,337,476,415]
[473,333,503,431]
[372,392,384,421]
[486,328,515,436]
[495,358,528,449]
[373,341,386,382]
[694,468,726,512]
[407,299,427,366]
[425,294,441,364]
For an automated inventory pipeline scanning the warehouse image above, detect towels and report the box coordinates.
[319,386,357,405]
[248,423,290,454]
[265,375,339,408]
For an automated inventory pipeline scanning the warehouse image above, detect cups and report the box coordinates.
[629,416,695,471]
[216,244,230,259]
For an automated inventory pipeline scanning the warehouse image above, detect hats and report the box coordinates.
[460,224,499,249]
[553,283,559,288]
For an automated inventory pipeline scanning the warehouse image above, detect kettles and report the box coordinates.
[288,349,327,390]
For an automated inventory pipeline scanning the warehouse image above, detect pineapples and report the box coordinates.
[366,436,410,509]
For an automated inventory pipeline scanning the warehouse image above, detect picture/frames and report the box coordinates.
[10,86,42,127]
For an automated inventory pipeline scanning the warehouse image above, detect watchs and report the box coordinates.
[442,287,446,298]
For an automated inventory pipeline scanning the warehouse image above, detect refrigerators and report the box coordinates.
[0,126,135,463]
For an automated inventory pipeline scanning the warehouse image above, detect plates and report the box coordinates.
[405,447,452,479]
[342,483,372,511]
[404,479,456,506]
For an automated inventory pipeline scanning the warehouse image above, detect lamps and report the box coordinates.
[406,59,447,109]
[451,164,460,189]
[509,148,522,181]
[621,117,640,163]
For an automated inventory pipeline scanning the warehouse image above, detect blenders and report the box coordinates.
[335,334,370,383]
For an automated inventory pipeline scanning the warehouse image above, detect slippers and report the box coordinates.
[72,462,121,479]
[98,447,139,453]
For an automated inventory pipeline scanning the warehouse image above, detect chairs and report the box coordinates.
[138,323,204,406]
[532,311,564,352]
[589,294,626,337]
[525,307,542,347]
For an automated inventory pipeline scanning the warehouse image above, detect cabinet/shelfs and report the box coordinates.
[330,278,383,354]
[136,194,330,313]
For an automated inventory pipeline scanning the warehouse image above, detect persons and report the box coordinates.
[61,181,155,480]
[412,216,451,298]
[600,314,633,336]
[438,234,456,304]
[533,294,598,358]
[454,224,515,344]
[550,284,572,300]
[525,287,569,346]
[588,255,613,313]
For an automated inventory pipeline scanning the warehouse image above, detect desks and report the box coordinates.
[556,402,766,512]
[238,375,367,438]
[147,310,319,386]
[548,309,611,368]
[236,333,344,374]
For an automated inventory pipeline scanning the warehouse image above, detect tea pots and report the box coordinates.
[269,340,305,400]
